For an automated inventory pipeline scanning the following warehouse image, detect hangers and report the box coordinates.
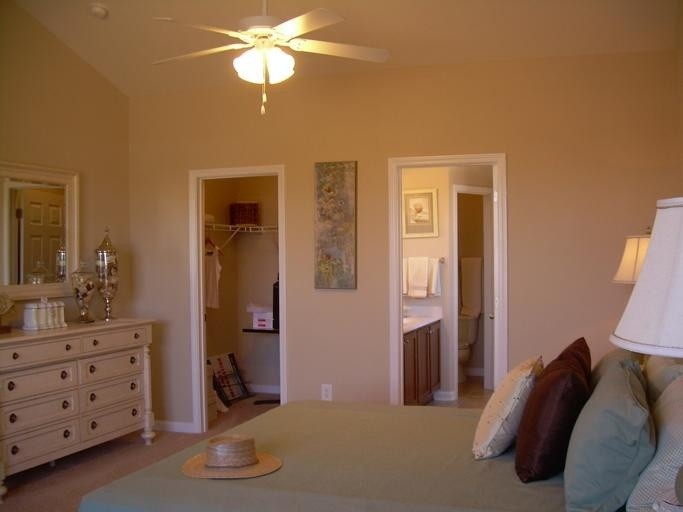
[204,234,225,258]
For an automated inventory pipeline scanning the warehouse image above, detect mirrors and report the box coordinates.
[0,162,82,305]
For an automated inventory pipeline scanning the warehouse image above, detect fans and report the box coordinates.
[148,0,390,66]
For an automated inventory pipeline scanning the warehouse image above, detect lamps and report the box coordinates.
[609,196,683,361]
[234,35,296,113]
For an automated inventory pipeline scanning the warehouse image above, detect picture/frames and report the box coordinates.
[401,188,439,240]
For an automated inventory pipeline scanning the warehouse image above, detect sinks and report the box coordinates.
[403,317,418,334]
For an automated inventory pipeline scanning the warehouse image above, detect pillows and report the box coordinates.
[469,334,683,512]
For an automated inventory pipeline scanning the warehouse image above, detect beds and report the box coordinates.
[80,398,565,512]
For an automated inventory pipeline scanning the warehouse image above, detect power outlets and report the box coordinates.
[320,384,334,402]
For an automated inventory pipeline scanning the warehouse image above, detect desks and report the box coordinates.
[241,327,280,405]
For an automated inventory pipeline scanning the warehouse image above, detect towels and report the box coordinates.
[402,256,442,298]
[461,258,484,320]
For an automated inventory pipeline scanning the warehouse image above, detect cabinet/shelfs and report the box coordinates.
[611,233,651,286]
[0,315,156,494]
[403,320,441,405]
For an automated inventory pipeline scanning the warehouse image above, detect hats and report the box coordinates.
[182,436,283,480]
